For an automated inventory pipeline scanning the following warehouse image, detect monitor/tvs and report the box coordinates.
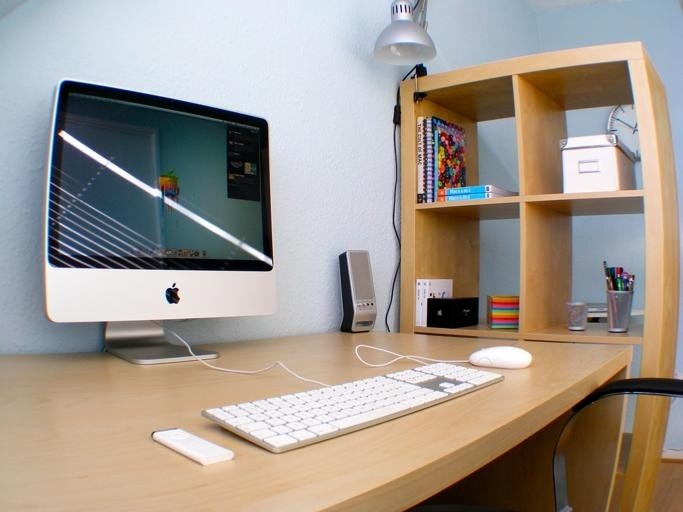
[42,78,278,366]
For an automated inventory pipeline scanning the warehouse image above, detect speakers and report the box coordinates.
[339,249,378,334]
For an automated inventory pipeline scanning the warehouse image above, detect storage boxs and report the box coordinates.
[488,296,519,327]
[560,133,636,193]
[427,297,479,327]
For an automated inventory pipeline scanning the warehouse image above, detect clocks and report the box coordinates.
[607,104,641,161]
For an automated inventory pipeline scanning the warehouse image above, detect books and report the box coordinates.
[415,116,516,205]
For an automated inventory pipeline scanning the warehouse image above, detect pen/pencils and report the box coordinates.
[604,261,635,329]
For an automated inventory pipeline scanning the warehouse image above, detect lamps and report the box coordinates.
[374,0,436,103]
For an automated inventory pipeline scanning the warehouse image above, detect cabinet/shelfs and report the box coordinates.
[399,40,683,511]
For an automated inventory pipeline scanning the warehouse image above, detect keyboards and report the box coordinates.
[200,361,505,454]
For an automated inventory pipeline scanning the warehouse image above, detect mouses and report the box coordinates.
[468,345,532,369]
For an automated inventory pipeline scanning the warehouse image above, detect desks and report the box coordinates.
[0,332,635,512]
